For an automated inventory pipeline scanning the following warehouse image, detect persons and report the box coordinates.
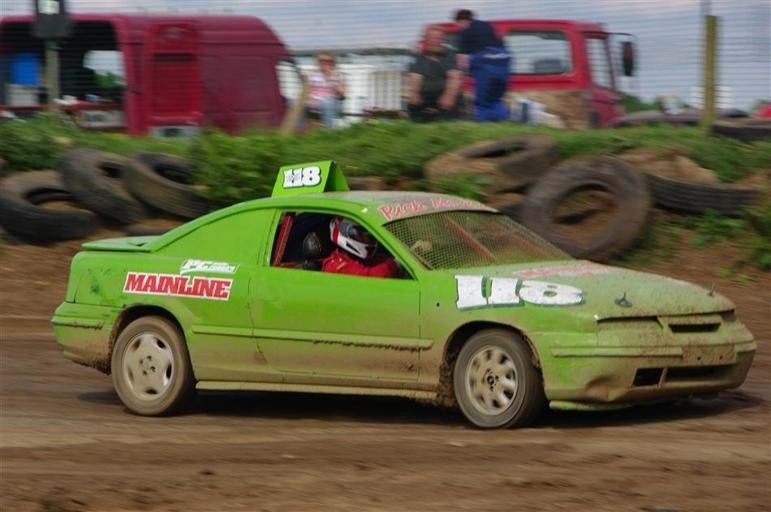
[453,9,513,122]
[293,51,348,129]
[321,215,432,278]
[406,26,466,122]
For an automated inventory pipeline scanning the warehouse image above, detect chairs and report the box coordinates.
[292,228,331,272]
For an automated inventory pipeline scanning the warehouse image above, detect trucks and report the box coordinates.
[421,15,639,122]
[3,10,322,133]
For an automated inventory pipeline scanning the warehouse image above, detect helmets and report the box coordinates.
[329,215,378,259]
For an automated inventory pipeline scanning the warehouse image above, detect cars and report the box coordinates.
[42,154,759,438]
